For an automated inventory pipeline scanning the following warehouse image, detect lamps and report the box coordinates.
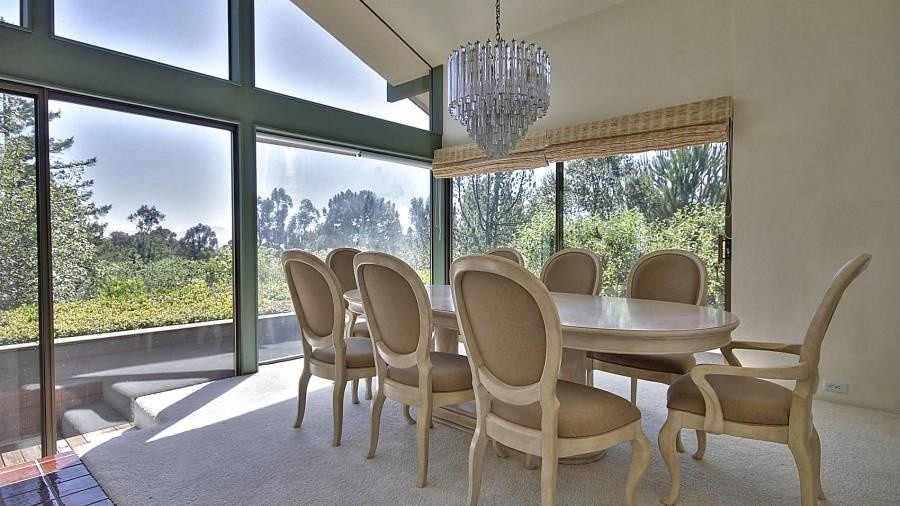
[443,0,552,161]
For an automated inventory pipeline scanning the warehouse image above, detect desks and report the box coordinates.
[339,283,741,471]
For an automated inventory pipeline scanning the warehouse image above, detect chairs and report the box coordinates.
[446,251,654,506]
[323,245,438,407]
[351,250,513,492]
[582,247,712,458]
[281,248,419,453]
[656,252,877,506]
[485,246,526,269]
[537,247,602,388]
